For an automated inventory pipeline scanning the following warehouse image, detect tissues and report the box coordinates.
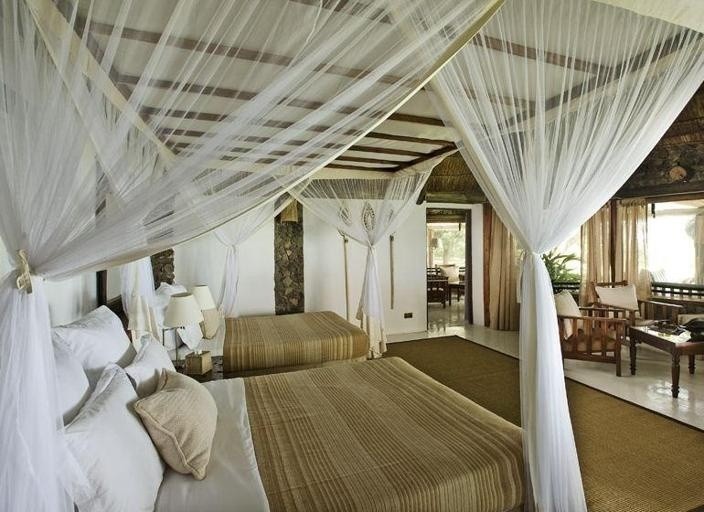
[185,349,214,375]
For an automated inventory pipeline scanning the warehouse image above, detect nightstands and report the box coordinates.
[169,357,224,382]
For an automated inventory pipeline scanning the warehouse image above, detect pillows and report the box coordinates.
[593,284,642,322]
[124,328,179,398]
[153,278,222,351]
[136,368,219,482]
[43,329,93,426]
[53,305,138,393]
[554,288,584,340]
[59,362,165,512]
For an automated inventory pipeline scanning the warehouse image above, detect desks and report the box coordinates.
[625,323,704,396]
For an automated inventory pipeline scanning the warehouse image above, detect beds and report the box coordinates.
[169,310,369,381]
[156,356,534,512]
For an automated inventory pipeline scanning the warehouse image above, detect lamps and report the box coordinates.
[162,291,204,372]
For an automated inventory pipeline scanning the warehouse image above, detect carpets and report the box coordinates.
[377,335,704,511]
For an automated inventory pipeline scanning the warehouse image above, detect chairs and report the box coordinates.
[556,306,626,380]
[428,262,467,308]
[587,279,685,358]
